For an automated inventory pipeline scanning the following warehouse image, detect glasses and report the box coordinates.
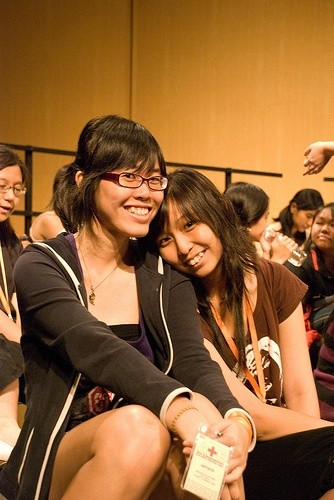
[0,184,27,197]
[100,171,168,191]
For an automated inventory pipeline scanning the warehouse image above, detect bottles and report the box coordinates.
[263,229,307,267]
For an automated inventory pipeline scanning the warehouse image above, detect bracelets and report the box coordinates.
[227,412,252,426]
[171,405,199,435]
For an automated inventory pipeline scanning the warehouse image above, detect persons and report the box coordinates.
[0,114,334,500]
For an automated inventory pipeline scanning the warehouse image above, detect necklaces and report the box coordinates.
[77,234,123,304]
